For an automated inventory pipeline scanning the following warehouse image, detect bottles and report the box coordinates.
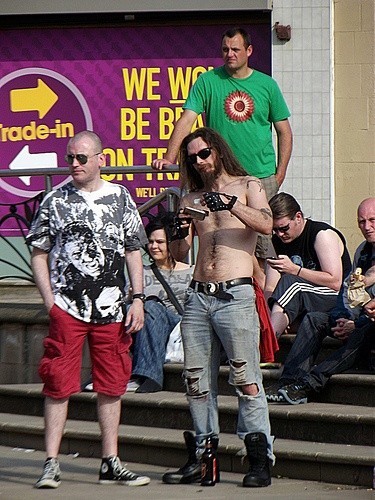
[201,436,216,486]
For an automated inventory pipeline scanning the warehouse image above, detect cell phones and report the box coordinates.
[182,207,206,221]
[266,257,277,260]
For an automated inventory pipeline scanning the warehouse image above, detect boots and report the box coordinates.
[242,432,272,487]
[162,430,221,484]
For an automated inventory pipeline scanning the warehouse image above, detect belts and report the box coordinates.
[187,276,255,303]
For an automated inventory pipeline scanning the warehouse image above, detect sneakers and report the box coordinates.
[98,456,151,487]
[277,378,308,405]
[265,381,287,402]
[34,456,62,488]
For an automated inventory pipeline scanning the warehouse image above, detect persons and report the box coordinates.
[25,130,151,490]
[258,192,353,367]
[161,127,276,488]
[151,27,293,316]
[263,197,375,405]
[85,219,195,392]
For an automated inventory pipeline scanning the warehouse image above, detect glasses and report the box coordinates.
[64,152,101,164]
[143,295,168,319]
[272,216,294,233]
[186,146,212,164]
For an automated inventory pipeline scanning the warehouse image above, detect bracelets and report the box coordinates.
[297,267,302,277]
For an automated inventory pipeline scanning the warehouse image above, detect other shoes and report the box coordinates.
[81,379,93,391]
[125,376,162,392]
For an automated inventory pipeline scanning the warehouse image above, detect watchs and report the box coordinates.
[132,293,147,304]
